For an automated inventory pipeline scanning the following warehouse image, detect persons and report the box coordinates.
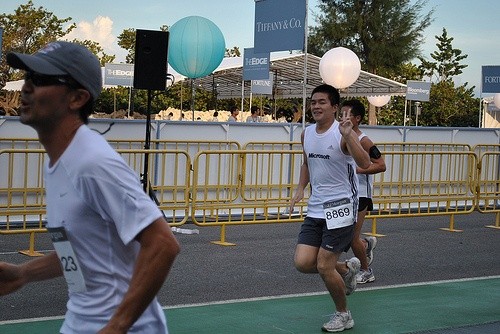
[166,105,294,124]
[290,84,371,331]
[338,100,386,283]
[0,42,181,334]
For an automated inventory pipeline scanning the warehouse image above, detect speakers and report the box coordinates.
[133,29,169,90]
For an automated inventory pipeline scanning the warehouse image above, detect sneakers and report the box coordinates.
[362,236,376,266]
[354,268,376,284]
[342,257,361,296]
[320,310,356,332]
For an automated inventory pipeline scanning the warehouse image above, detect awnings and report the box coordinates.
[3,80,25,91]
[167,53,407,123]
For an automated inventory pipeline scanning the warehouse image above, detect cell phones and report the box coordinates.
[370,146,381,159]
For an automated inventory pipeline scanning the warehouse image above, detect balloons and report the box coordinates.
[168,16,225,80]
[319,47,360,89]
[367,95,390,108]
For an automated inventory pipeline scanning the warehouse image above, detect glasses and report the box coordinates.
[23,71,75,88]
[338,113,355,119]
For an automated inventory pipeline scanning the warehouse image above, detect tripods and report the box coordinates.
[140,89,169,222]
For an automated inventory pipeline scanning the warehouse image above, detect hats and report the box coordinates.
[5,41,105,103]
